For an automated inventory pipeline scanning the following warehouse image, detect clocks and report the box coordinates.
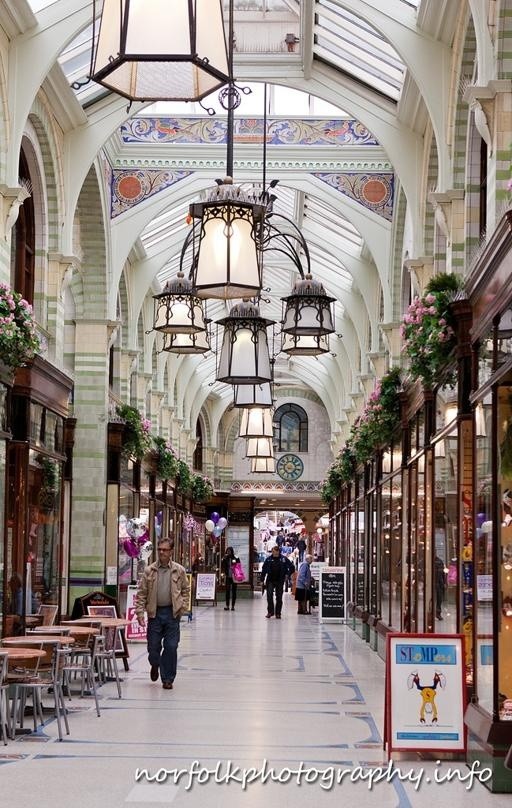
[276,453,304,482]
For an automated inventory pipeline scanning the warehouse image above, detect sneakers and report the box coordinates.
[267,612,273,618]
[276,614,280,618]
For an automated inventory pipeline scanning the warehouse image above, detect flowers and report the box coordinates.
[116,404,155,458]
[0,281,44,378]
[319,364,402,503]
[402,272,459,378]
[153,437,218,498]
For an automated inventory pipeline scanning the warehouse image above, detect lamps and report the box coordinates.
[189,0,267,299]
[68,1,251,115]
[228,358,279,474]
[146,212,343,383]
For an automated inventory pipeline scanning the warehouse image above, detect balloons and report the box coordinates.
[119,512,228,563]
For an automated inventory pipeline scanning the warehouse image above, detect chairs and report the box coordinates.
[0,613,133,746]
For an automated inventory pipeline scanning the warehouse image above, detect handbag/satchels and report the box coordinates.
[231,572,245,584]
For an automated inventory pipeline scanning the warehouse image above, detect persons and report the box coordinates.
[133,537,193,692]
[432,546,448,622]
[222,515,315,621]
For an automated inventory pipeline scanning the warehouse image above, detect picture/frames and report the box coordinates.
[38,605,59,626]
[87,605,125,653]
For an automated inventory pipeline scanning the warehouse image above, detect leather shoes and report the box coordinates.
[151,664,158,681]
[163,682,173,688]
